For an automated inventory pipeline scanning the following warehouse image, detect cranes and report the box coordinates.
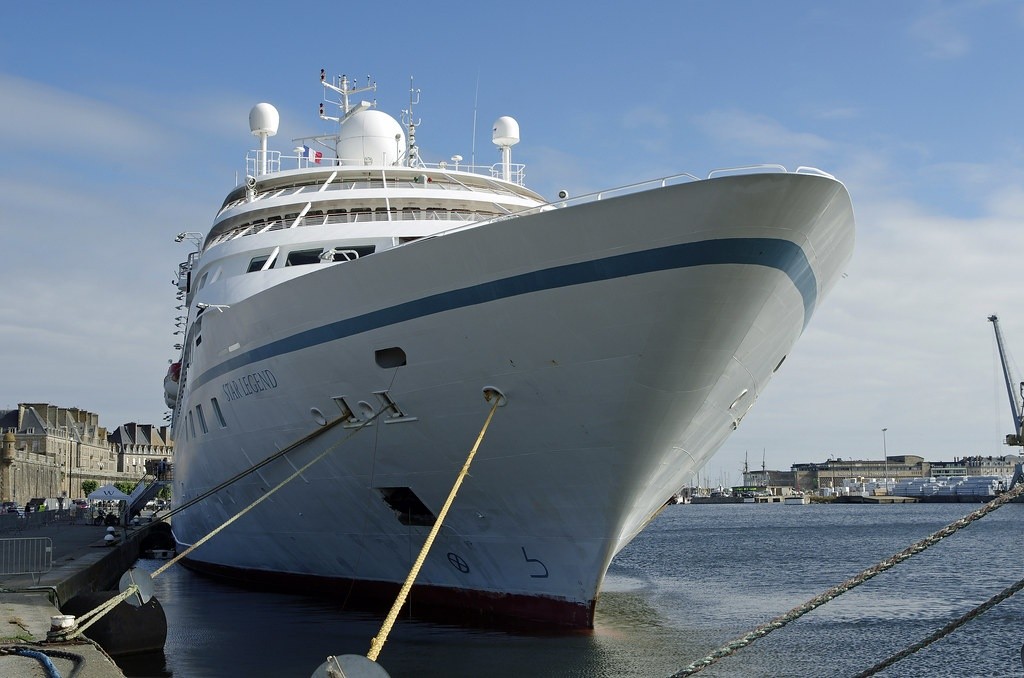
[988,314,1024,490]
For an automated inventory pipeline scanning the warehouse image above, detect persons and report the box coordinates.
[69,500,77,525]
[154,457,172,481]
[34,503,39,511]
[25,502,30,524]
[80,508,84,519]
[2,502,17,515]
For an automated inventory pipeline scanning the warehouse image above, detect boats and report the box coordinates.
[162,65,856,638]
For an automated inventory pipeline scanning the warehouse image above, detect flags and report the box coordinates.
[302,144,323,164]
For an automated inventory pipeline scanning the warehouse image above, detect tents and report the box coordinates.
[87,483,131,527]
